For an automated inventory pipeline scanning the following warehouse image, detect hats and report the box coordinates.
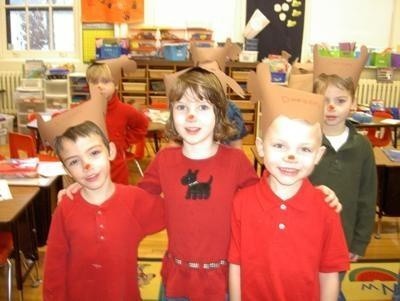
[190,43,229,71]
[37,93,108,150]
[164,62,227,112]
[247,64,325,139]
[312,45,369,94]
[92,55,137,86]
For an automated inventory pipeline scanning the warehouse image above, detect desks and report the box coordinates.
[249,145,265,178]
[0,186,43,301]
[147,120,166,154]
[352,118,400,148]
[0,161,63,219]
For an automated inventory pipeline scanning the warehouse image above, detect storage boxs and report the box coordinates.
[163,43,188,61]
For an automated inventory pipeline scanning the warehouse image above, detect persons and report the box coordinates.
[36,85,168,301]
[86,63,150,186]
[228,63,350,301]
[308,44,377,262]
[56,68,343,301]
[169,42,248,149]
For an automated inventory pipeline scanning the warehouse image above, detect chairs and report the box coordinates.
[125,134,145,179]
[0,231,14,301]
[27,113,38,144]
[9,132,37,160]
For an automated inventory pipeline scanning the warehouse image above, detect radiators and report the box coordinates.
[355,78,400,108]
[0,71,22,115]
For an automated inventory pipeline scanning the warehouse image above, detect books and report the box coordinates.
[380,146,400,162]
[0,169,39,184]
[0,157,40,169]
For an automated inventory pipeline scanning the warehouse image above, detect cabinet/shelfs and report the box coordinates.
[90,60,262,181]
[15,74,90,132]
